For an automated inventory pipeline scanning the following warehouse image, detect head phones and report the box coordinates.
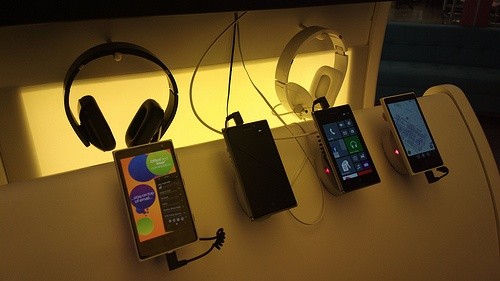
[63,41,180,153]
[275,27,348,121]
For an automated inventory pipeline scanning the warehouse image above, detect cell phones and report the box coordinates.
[112,140,199,261]
[380,92,445,176]
[311,104,382,195]
[221,121,297,220]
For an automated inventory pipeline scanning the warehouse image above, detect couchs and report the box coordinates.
[376,22,500,113]
